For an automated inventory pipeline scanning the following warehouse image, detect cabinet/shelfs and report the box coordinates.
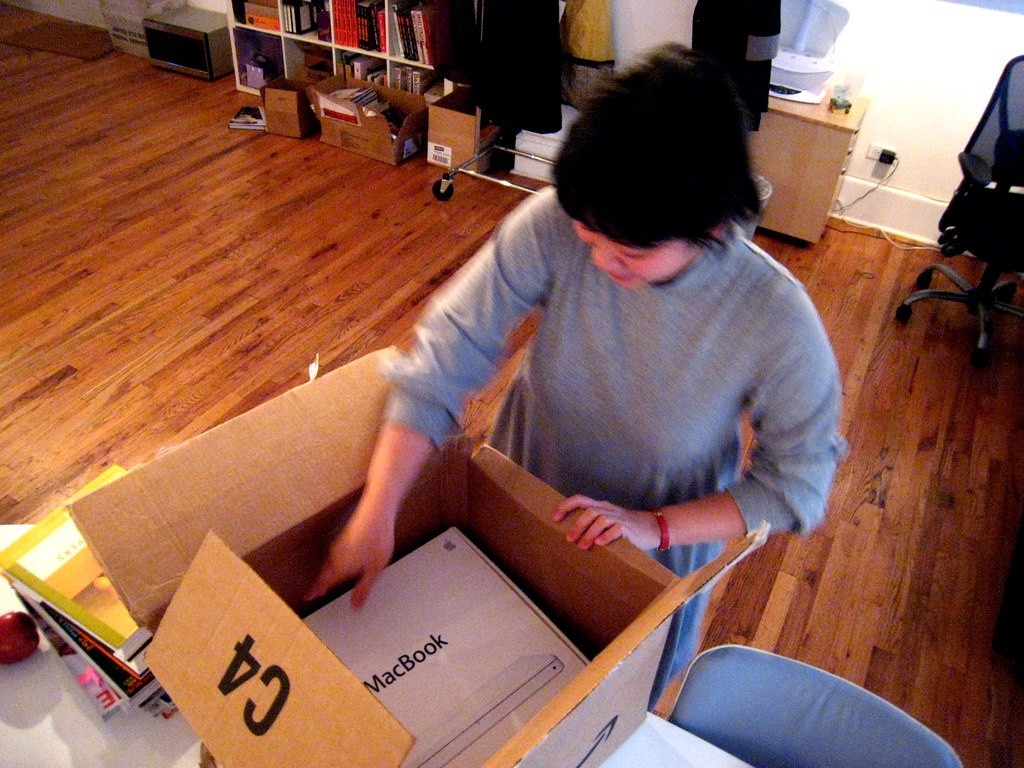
[226,0,560,110]
[747,89,869,244]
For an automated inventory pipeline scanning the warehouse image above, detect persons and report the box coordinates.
[302,43,852,712]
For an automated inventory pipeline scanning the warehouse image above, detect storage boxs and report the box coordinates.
[259,67,502,175]
[68,341,771,768]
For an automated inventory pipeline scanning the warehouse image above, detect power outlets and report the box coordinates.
[866,144,897,164]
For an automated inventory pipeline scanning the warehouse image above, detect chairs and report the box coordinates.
[668,645,965,768]
[895,56,1024,367]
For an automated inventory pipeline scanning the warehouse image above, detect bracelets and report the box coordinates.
[652,509,670,553]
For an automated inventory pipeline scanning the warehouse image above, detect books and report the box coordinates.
[0,463,178,719]
[231,0,439,130]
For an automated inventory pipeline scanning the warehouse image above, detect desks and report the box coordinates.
[0,525,757,768]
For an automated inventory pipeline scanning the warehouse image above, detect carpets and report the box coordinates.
[0,10,114,61]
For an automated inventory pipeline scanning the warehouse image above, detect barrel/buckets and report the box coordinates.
[738,173,773,242]
